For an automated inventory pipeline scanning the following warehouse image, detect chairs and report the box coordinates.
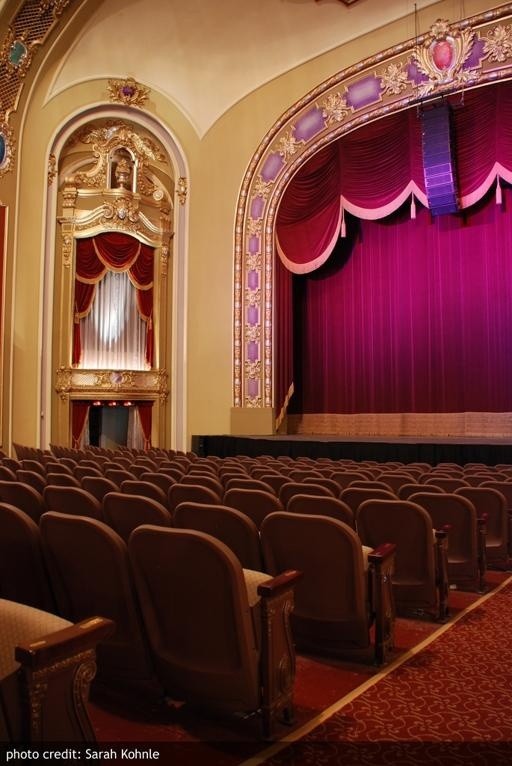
[2,439,511,765]
